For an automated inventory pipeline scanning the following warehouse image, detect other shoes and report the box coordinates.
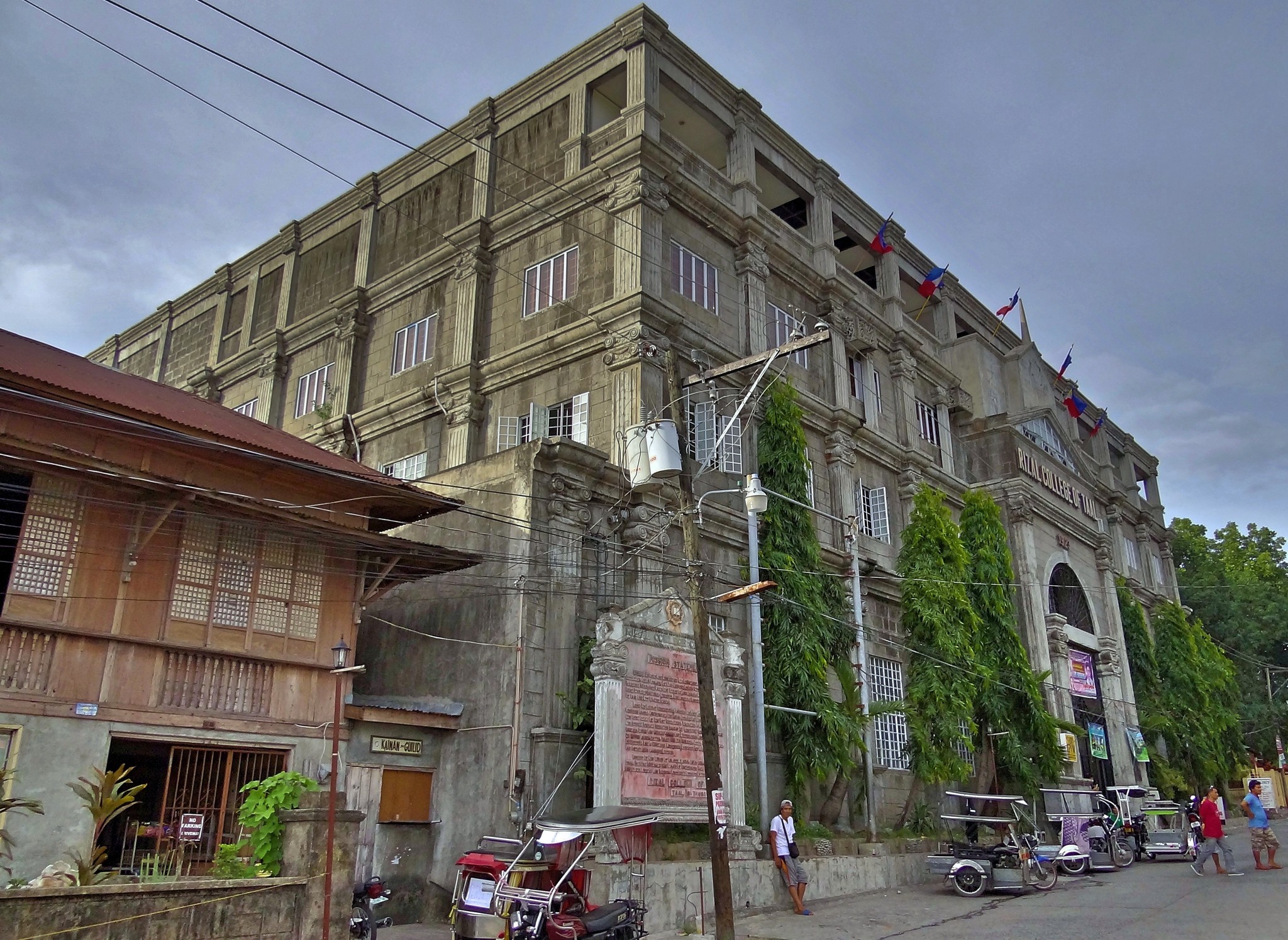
[1228,871,1244,876]
[1191,864,1204,876]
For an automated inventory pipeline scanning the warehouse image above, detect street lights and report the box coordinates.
[681,474,768,940]
[986,721,1004,844]
[322,636,352,940]
[1181,733,1200,801]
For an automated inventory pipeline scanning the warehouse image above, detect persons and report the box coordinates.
[1198,787,1227,874]
[1070,676,1075,691]
[770,800,813,915]
[1107,805,1122,828]
[1084,661,1094,686]
[1190,785,1244,876]
[1240,779,1283,870]
[1092,737,1096,749]
[1086,775,1098,791]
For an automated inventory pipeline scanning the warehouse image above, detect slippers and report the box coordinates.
[1269,864,1282,869]
[1216,869,1227,874]
[1254,866,1270,870]
[796,908,813,915]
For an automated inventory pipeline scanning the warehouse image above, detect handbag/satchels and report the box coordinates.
[788,843,800,859]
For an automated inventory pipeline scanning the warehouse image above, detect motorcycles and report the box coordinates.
[1030,784,1205,876]
[925,791,1057,898]
[349,876,393,940]
[450,805,665,940]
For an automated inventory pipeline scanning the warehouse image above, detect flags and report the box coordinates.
[870,211,894,253]
[1063,388,1087,418]
[1056,348,1073,380]
[1089,418,1103,437]
[918,265,948,297]
[996,288,1019,316]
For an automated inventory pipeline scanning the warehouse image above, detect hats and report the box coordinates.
[780,800,792,808]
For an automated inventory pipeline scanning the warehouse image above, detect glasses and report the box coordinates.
[1210,786,1214,789]
